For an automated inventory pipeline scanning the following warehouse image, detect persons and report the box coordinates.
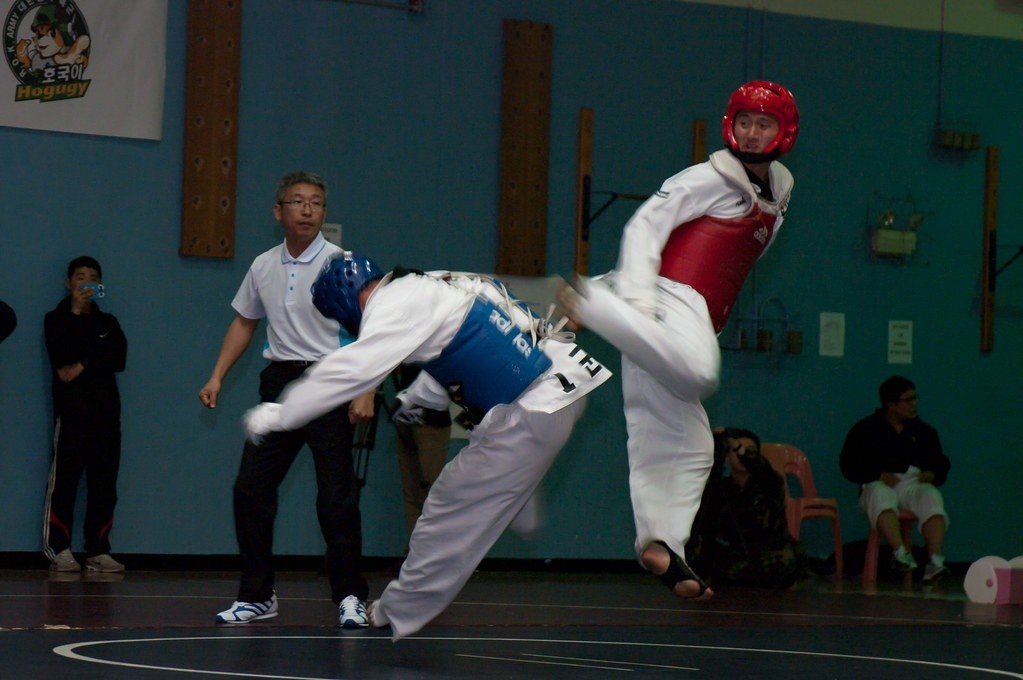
[42,255,127,573]
[198,173,378,629]
[555,82,798,600]
[839,376,964,594]
[686,426,798,592]
[237,251,614,640]
[394,407,451,532]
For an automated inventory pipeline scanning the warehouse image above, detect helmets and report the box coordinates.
[722,81,799,160]
[313,253,385,337]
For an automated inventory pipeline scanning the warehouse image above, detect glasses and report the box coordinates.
[896,394,918,402]
[278,201,326,212]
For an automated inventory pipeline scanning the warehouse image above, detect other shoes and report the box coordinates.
[892,553,917,571]
[923,565,948,586]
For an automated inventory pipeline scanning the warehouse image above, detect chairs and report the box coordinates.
[756,441,839,579]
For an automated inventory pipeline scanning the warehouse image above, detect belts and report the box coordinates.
[271,360,316,367]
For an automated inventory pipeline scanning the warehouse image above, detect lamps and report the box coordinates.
[869,211,925,258]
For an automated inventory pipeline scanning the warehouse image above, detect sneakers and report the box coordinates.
[338,596,368,627]
[86,554,124,572]
[216,589,278,624]
[50,548,81,571]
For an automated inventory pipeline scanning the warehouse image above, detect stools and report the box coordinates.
[862,509,918,588]
